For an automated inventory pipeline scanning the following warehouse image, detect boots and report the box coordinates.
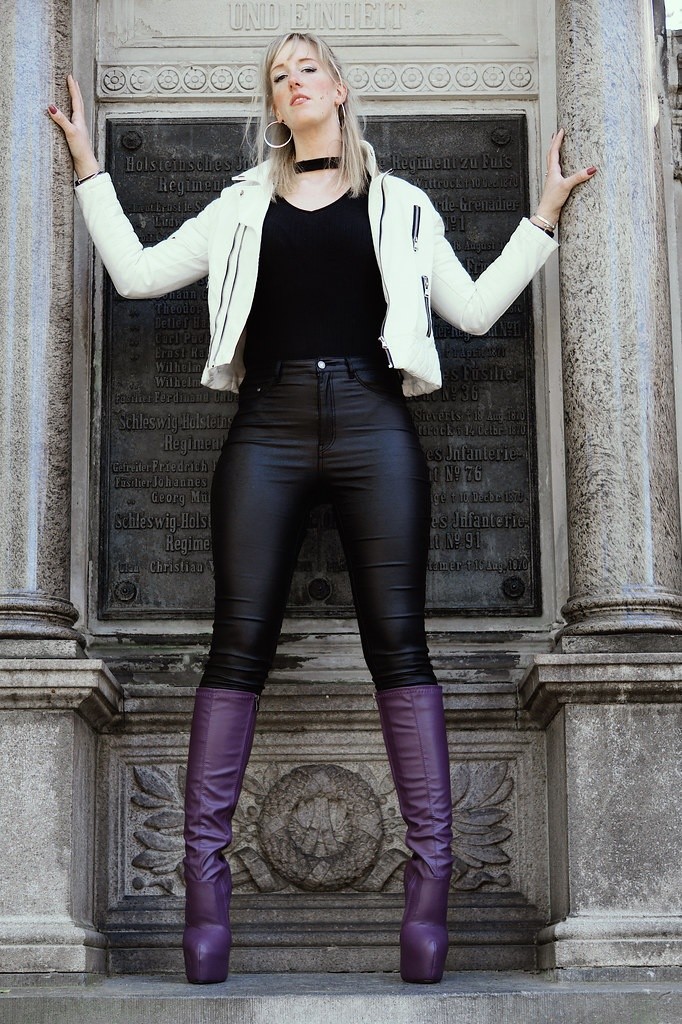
[181,690,260,986]
[377,684,453,983]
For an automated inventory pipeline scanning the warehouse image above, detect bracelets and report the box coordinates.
[75,168,107,187]
[533,211,556,231]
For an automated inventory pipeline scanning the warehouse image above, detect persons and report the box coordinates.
[45,27,596,988]
